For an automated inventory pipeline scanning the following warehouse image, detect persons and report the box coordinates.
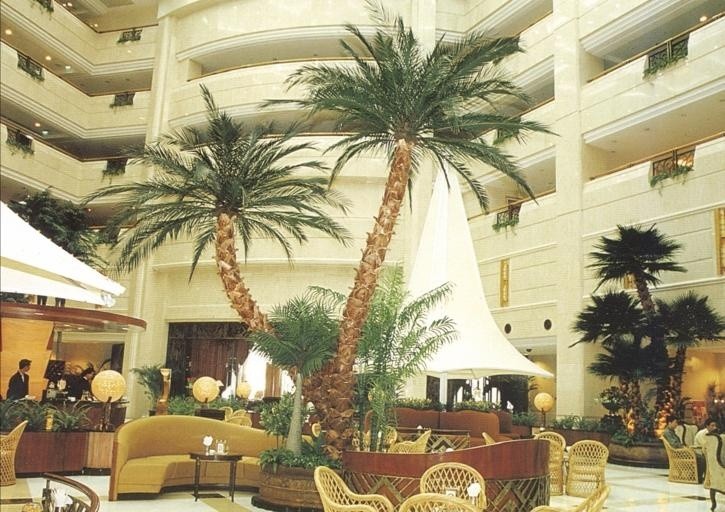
[663,416,705,483]
[694,418,719,476]
[77,367,95,400]
[7,359,32,400]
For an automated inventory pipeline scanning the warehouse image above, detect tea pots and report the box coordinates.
[81,389,90,401]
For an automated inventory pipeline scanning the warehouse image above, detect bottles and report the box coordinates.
[218,440,224,454]
[42,480,53,510]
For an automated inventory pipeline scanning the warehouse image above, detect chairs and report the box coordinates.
[400,493,476,510]
[660,432,699,483]
[481,432,495,446]
[564,440,610,498]
[675,422,706,478]
[531,484,610,510]
[703,433,725,511]
[419,462,487,509]
[533,432,565,497]
[0,419,29,486]
[387,430,432,453]
[314,466,395,512]
[41,472,101,512]
[366,425,398,452]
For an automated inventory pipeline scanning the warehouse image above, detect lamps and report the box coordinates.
[91,370,125,430]
[236,379,250,399]
[533,392,555,427]
[190,376,219,408]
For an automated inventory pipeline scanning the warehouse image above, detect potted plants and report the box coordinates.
[129,364,163,416]
[71,6,562,511]
[568,221,725,470]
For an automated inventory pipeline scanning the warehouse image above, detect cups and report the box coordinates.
[22,502,43,512]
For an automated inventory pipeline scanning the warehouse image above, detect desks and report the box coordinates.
[189,449,245,503]
[84,428,115,477]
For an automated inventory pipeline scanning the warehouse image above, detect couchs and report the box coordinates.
[108,414,313,503]
[364,406,521,446]
